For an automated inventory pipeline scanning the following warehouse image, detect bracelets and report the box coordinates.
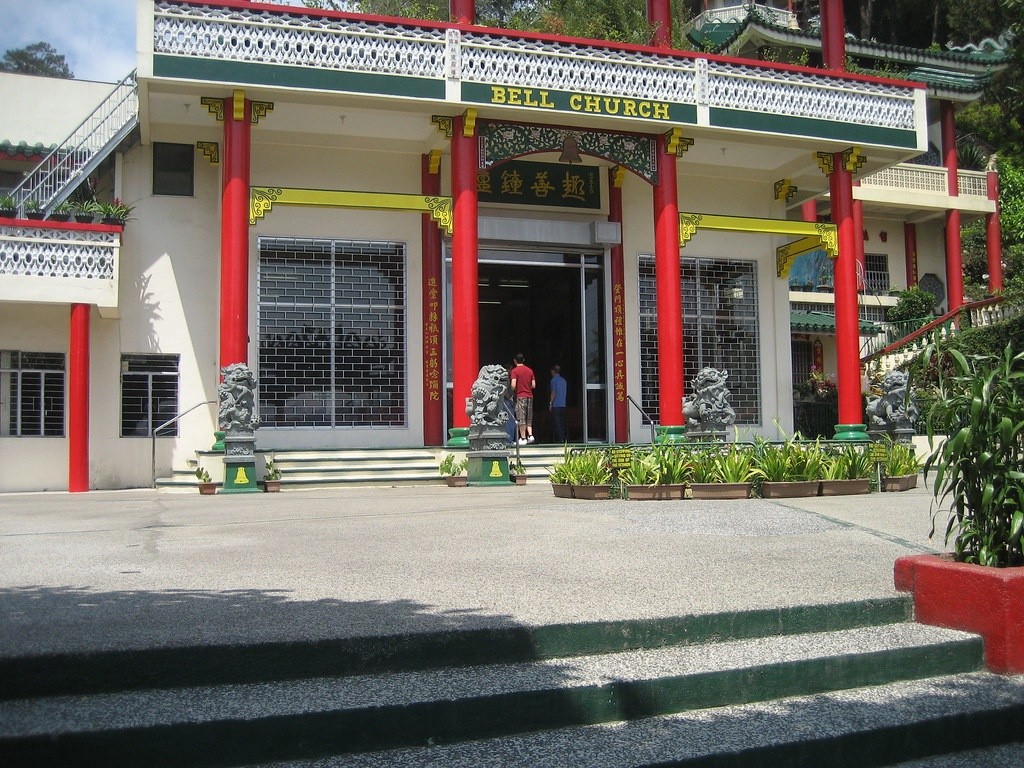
[550,402,552,405]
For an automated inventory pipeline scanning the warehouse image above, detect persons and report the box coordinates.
[549,365,568,442]
[499,354,536,445]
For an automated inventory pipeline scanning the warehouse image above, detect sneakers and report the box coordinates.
[514,438,527,445]
[527,436,535,444]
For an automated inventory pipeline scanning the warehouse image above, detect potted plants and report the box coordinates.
[94,196,140,227]
[508,460,527,484]
[194,466,216,494]
[439,452,469,487]
[547,420,927,498]
[51,199,75,221]
[23,198,47,220]
[75,201,96,222]
[789,266,901,297]
[263,461,283,491]
[0,191,19,218]
